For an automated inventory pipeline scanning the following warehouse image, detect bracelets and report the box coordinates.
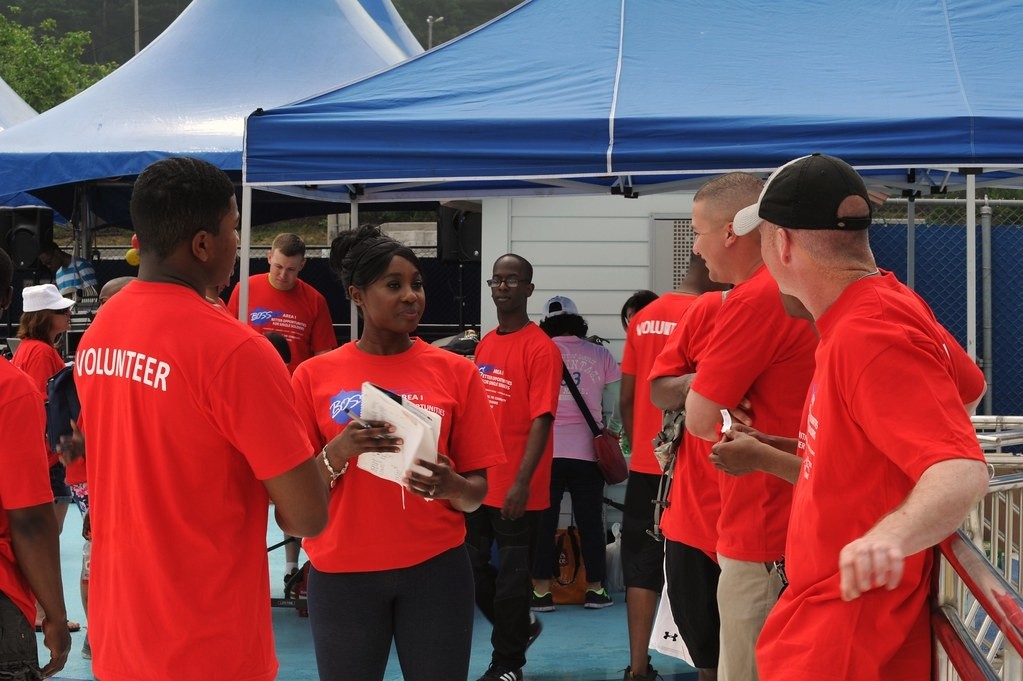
[323,444,349,488]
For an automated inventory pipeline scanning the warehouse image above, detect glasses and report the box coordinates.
[49,308,68,314]
[487,279,530,288]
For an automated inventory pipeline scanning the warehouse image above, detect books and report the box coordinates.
[357,381,442,491]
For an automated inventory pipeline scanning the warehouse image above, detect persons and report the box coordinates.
[0,244,136,681]
[72,158,329,680]
[474,252,564,681]
[291,224,510,681]
[693,173,817,681]
[646,290,733,681]
[531,296,622,612]
[709,153,987,681]
[619,255,731,681]
[228,233,339,602]
[621,290,658,333]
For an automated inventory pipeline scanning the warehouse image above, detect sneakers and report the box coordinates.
[528,588,555,612]
[82,625,92,660]
[476,662,523,681]
[524,609,542,652]
[283,568,302,599]
[618,655,663,681]
[585,587,614,609]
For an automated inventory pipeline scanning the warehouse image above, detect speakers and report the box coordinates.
[0,205,53,271]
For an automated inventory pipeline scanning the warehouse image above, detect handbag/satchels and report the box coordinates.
[532,523,587,605]
[604,522,628,593]
[651,540,696,669]
[654,403,685,479]
[592,425,628,485]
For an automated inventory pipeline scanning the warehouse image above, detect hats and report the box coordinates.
[732,153,872,237]
[22,284,75,312]
[540,296,578,320]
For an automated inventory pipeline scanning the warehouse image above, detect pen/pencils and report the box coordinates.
[344,407,388,439]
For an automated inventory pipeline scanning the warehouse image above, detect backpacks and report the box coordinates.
[41,363,84,454]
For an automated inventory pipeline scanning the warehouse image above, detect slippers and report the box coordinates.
[33,618,79,633]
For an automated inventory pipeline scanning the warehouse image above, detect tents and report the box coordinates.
[240,0,1022,411]
[1,0,425,344]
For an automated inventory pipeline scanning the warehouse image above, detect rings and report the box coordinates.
[429,485,435,495]
